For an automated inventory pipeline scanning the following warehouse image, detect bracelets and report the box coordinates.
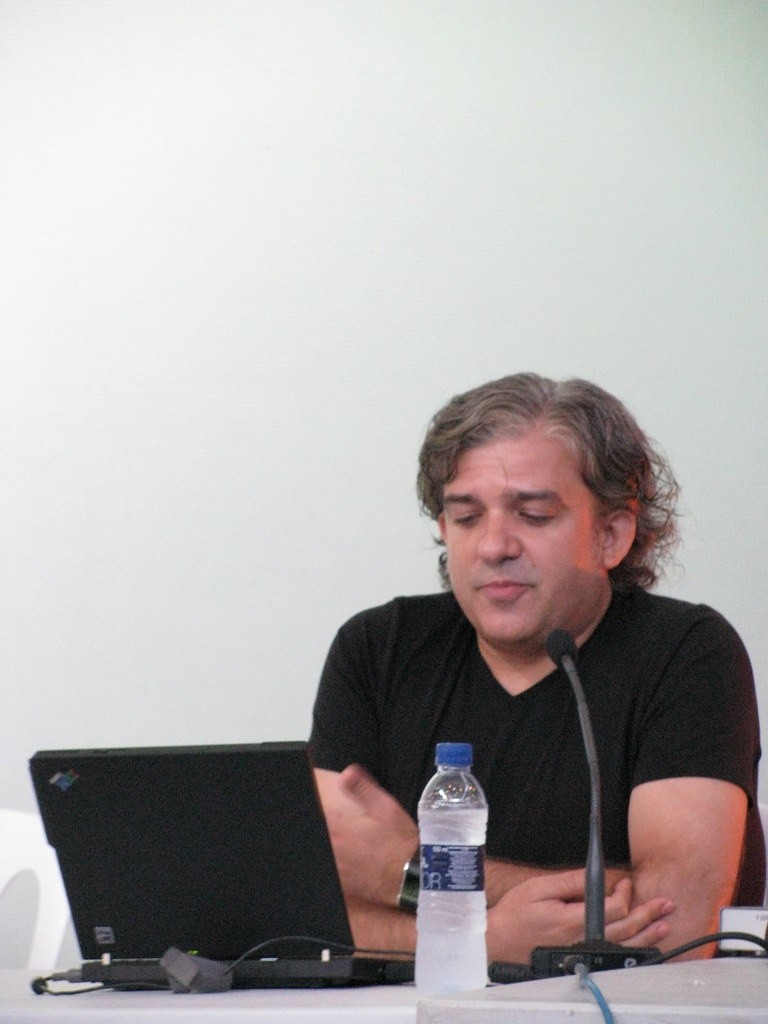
[396,847,421,916]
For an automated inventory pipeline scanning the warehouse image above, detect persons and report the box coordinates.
[308,372,767,971]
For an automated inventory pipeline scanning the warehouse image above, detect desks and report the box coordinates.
[0,968,417,1024]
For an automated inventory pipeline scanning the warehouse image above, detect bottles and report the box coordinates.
[414,743,490,1001]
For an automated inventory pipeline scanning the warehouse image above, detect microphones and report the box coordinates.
[528,628,664,984]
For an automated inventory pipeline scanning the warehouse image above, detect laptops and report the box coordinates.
[28,740,418,996]
